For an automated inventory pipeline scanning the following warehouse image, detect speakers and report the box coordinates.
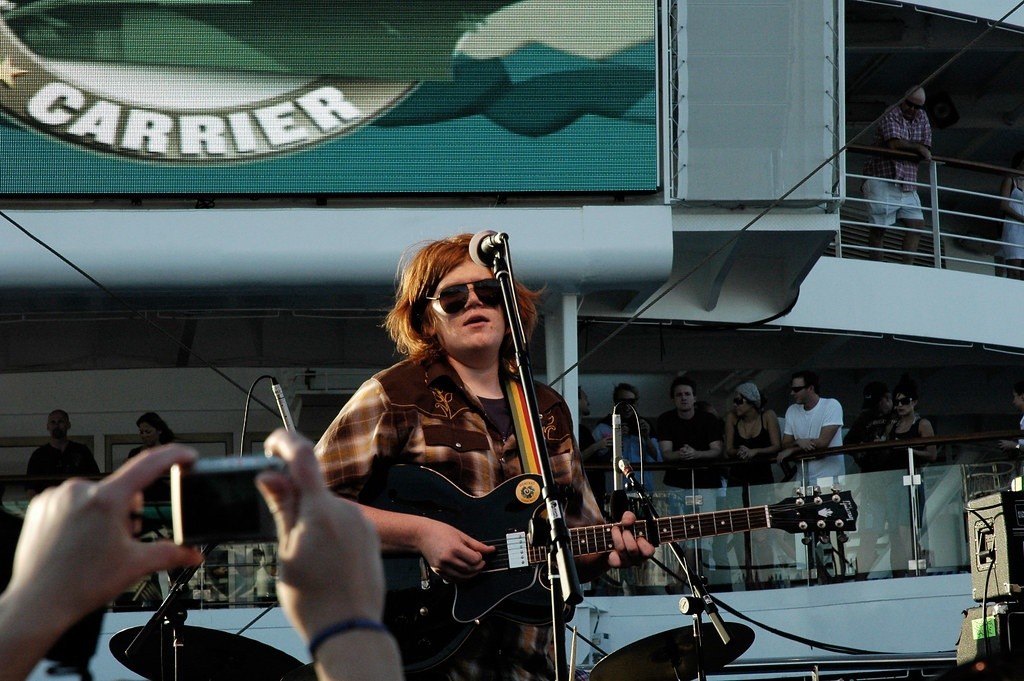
[956,605,1024,681]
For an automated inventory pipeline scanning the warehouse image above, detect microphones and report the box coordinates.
[610,406,629,522]
[271,376,298,435]
[469,230,510,267]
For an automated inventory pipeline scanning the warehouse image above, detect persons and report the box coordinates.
[578,386,612,495]
[873,372,938,470]
[998,380,1024,475]
[777,371,845,485]
[656,376,724,491]
[313,235,655,681]
[26,409,100,475]
[843,381,899,473]
[860,86,931,264]
[0,428,403,681]
[1000,154,1024,281]
[123,412,175,465]
[593,382,662,492]
[724,383,781,486]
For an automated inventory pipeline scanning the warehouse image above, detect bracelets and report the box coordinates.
[1015,444,1019,450]
[309,617,389,660]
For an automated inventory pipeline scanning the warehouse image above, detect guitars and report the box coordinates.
[325,460,859,675]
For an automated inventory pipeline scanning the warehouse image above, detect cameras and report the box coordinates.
[169,454,296,546]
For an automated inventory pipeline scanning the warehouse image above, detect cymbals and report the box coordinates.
[106,624,319,681]
[587,621,756,681]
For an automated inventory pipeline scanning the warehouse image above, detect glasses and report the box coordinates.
[426,278,503,314]
[792,385,807,392]
[892,397,912,406]
[733,398,747,405]
[618,398,636,405]
